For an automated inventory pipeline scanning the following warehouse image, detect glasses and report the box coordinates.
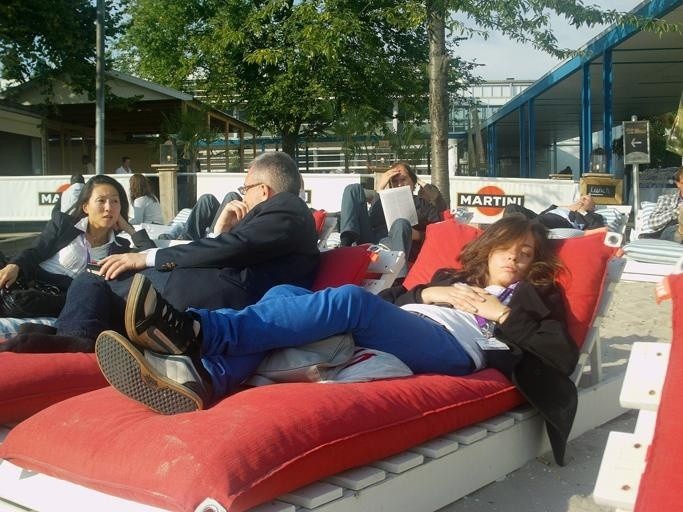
[237,182,262,194]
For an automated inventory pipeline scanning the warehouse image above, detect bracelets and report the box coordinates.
[496,308,511,324]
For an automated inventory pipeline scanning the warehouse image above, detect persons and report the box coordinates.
[503,194,604,230]
[94,212,579,416]
[364,177,446,217]
[0,175,157,318]
[158,222,185,240]
[0,150,323,354]
[179,192,242,240]
[125,174,164,226]
[51,174,85,218]
[115,156,132,174]
[339,163,441,287]
[81,155,94,174]
[637,169,682,244]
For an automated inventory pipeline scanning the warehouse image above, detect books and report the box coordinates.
[367,249,405,274]
[362,258,405,295]
[377,185,418,233]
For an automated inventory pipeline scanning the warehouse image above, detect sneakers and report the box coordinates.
[94,330,204,417]
[124,272,201,358]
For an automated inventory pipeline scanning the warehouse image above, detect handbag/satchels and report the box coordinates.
[0,276,67,318]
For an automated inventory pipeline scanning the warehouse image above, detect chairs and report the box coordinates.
[593,271,682,509]
[619,199,683,285]
[314,209,340,250]
[133,209,196,241]
[366,210,475,277]
[2,244,405,431]
[3,221,639,509]
[547,205,633,247]
